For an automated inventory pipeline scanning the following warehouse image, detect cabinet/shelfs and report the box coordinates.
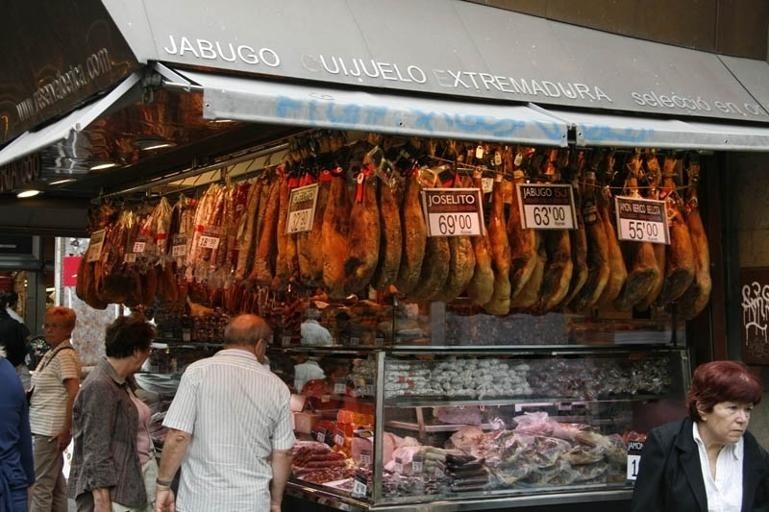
[135,343,692,512]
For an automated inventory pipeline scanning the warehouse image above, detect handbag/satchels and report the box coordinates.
[112,459,159,512]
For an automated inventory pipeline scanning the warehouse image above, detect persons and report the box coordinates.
[125,372,160,511]
[0,291,9,359]
[66,314,154,511]
[293,356,328,393]
[25,305,83,510]
[6,289,35,394]
[335,311,367,346]
[156,316,185,339]
[1,355,38,510]
[302,353,353,396]
[297,308,335,349]
[154,313,296,511]
[627,358,769,511]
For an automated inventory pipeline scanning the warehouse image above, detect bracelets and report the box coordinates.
[155,483,171,491]
[157,478,172,487]
